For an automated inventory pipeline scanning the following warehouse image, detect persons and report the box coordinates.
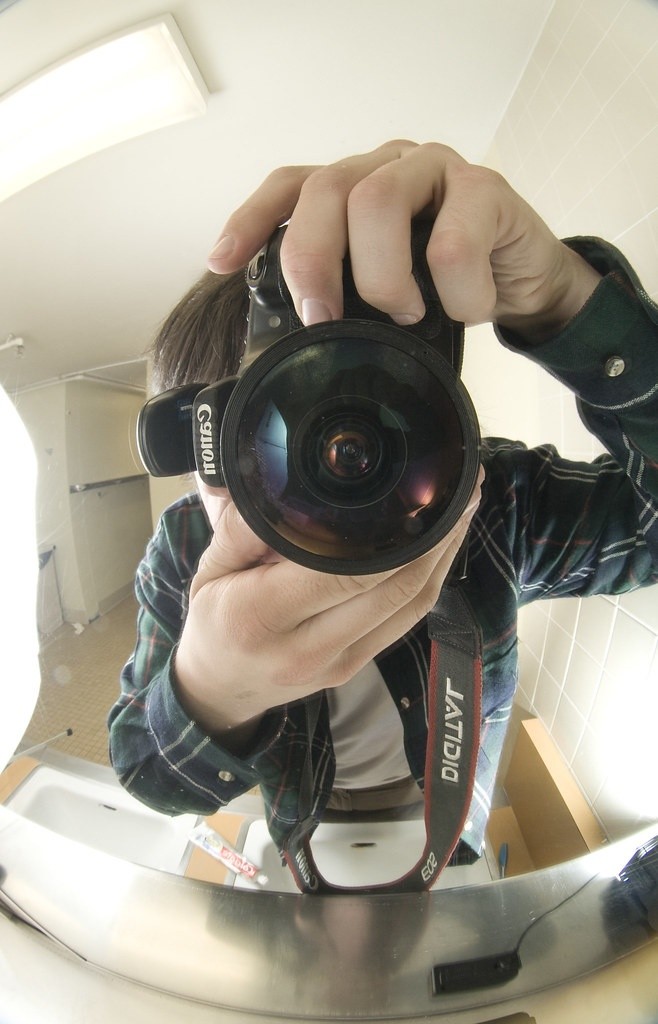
[107,139,658,896]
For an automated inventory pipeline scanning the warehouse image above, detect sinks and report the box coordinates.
[7,762,206,876]
[234,812,496,891]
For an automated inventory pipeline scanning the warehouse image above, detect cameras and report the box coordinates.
[134,222,483,576]
